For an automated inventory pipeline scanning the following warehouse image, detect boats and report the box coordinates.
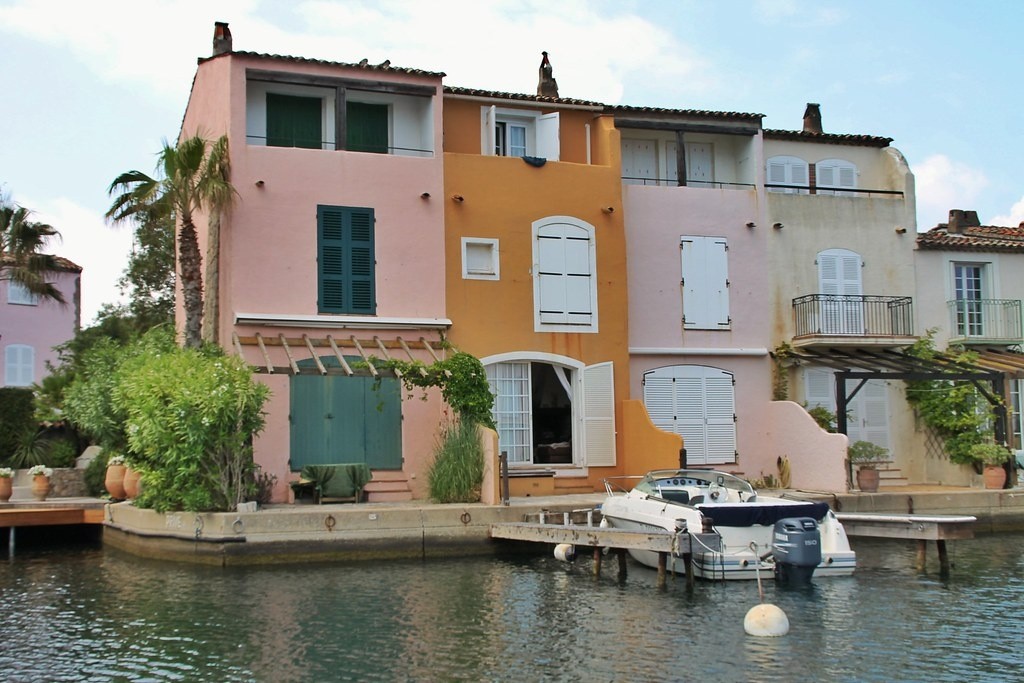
[601,467,858,579]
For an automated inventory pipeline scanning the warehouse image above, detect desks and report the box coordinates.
[302,463,369,504]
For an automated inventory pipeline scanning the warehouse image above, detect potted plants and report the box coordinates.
[846,440,889,491]
[123,461,153,499]
[969,443,1012,491]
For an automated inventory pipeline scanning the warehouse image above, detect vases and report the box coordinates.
[0,476,13,502]
[105,465,128,501]
[31,474,52,501]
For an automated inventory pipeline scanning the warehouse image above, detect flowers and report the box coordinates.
[105,455,129,469]
[0,466,15,480]
[27,464,53,477]
